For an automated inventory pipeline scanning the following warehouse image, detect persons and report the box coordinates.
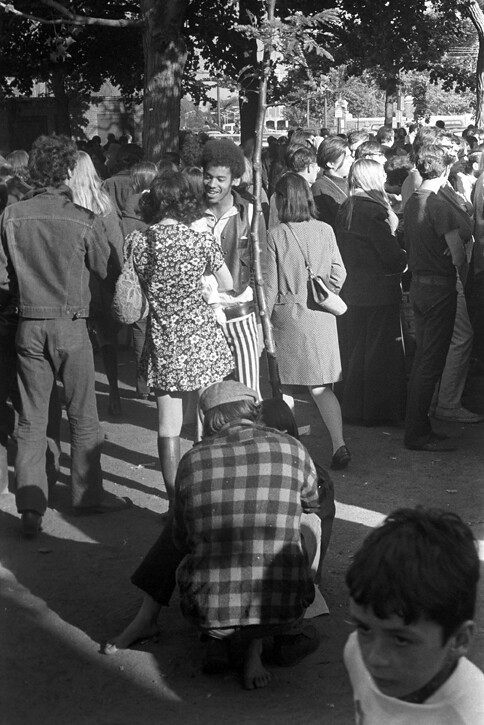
[263,172,352,470]
[403,144,473,452]
[0,120,484,492]
[343,504,484,725]
[105,397,335,689]
[334,158,408,425]
[174,380,323,668]
[123,170,237,524]
[191,139,265,407]
[0,135,133,535]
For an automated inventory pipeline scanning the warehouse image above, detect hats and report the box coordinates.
[200,381,259,413]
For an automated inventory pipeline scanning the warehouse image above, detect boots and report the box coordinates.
[101,344,122,416]
[158,435,181,522]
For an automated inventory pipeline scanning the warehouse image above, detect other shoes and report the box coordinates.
[275,624,320,667]
[20,511,42,536]
[136,392,150,400]
[420,437,457,452]
[330,445,350,469]
[72,491,132,516]
[205,642,231,675]
[434,430,449,441]
[435,404,481,423]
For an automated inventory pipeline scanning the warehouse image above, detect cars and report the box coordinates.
[203,122,241,147]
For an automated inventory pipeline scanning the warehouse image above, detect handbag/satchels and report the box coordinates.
[108,231,149,324]
[309,275,348,314]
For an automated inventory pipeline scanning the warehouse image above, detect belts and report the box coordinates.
[224,302,256,321]
